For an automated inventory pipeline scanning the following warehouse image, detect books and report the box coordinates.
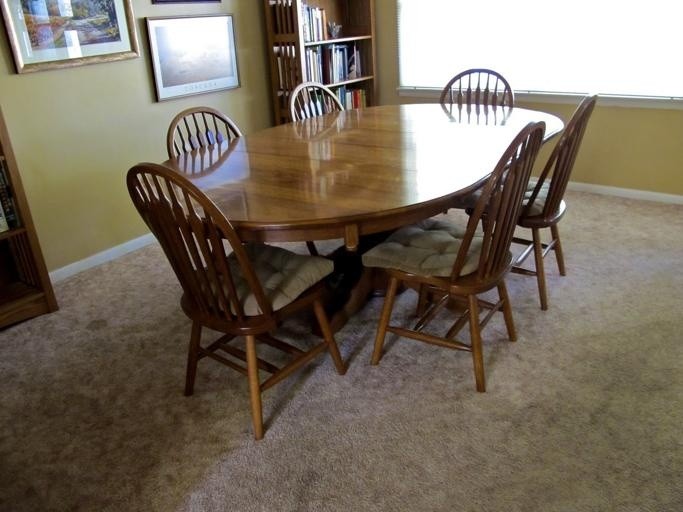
[271,0,367,113]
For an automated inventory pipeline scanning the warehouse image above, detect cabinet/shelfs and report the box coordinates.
[0,107,60,336]
[264,0,377,127]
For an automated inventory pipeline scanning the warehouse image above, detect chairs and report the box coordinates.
[291,81,343,124]
[439,69,515,108]
[166,106,242,159]
[467,92,598,310]
[441,103,512,126]
[358,120,547,393]
[126,162,345,441]
[171,136,241,179]
[290,112,341,143]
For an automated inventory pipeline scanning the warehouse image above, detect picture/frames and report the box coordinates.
[152,0,221,4]
[0,0,141,74]
[144,13,241,101]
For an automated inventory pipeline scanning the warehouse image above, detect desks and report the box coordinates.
[144,102,565,336]
